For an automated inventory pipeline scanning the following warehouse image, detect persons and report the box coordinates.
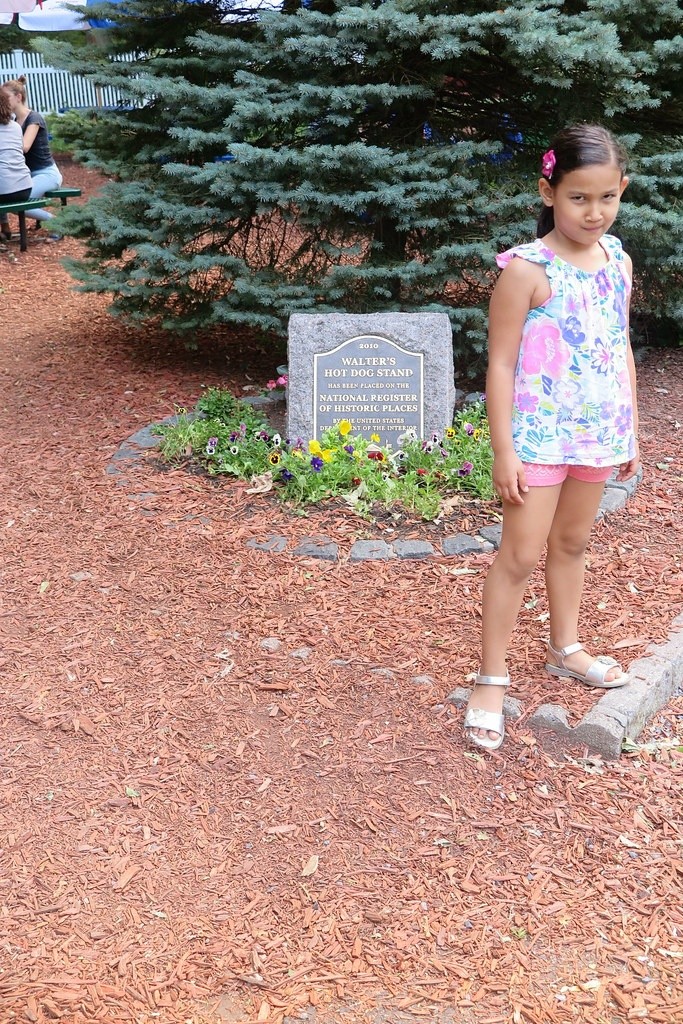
[460,121,640,752]
[0,74,63,243]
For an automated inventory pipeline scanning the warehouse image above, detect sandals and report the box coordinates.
[464,668,510,749]
[545,641,629,688]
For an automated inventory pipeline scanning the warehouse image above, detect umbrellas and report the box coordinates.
[18,0,313,33]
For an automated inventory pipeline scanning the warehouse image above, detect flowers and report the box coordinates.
[542,150,556,180]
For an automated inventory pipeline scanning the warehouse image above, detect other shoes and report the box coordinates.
[46,224,62,243]
[0,231,12,240]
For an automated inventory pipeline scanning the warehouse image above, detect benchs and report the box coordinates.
[44,187,82,207]
[0,195,53,252]
